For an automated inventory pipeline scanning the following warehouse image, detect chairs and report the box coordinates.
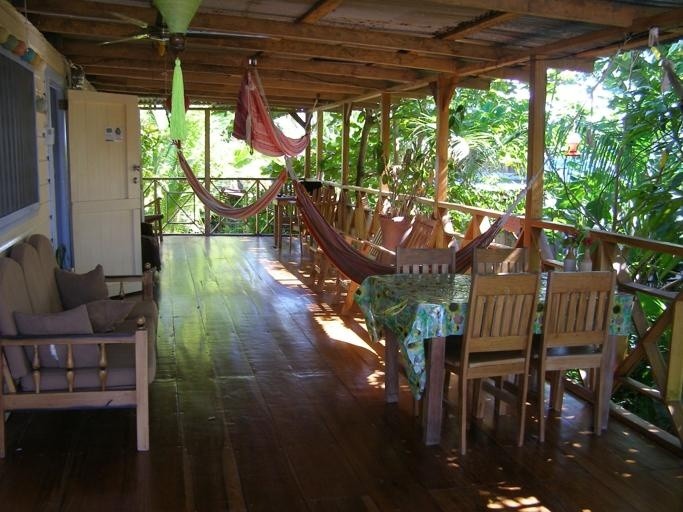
[309,212,445,314]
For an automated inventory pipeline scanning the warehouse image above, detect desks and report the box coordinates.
[273,196,324,254]
[368,272,635,447]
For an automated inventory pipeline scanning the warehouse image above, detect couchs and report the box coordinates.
[1,233,159,458]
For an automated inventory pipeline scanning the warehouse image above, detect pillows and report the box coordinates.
[13,263,138,368]
